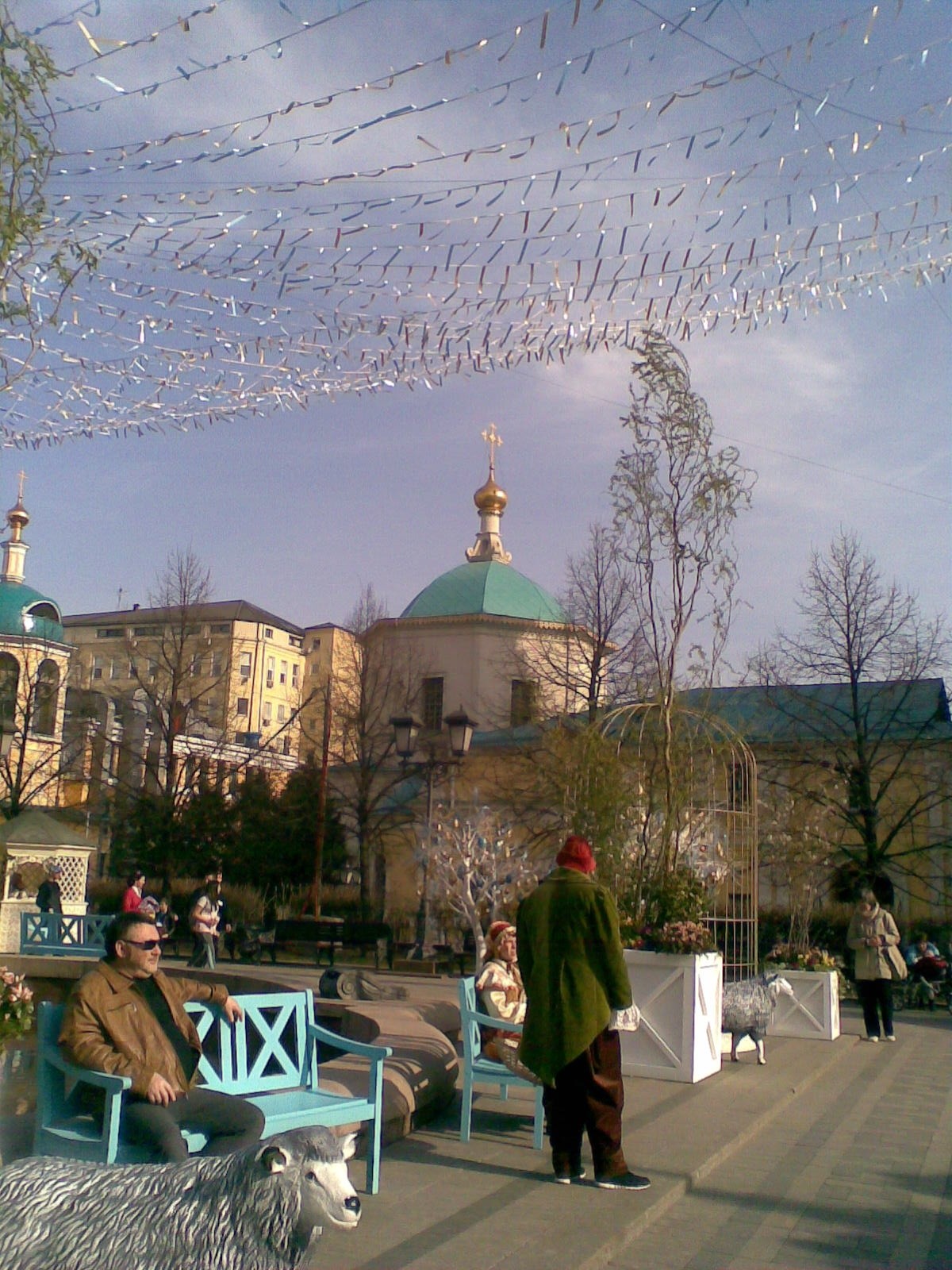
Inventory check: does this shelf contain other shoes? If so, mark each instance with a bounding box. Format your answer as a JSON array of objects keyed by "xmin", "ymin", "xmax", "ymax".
[
  {"xmin": 594, "ymin": 1170, "xmax": 651, "ymax": 1190},
  {"xmin": 868, "ymin": 1035, "xmax": 879, "ymax": 1043},
  {"xmin": 887, "ymin": 1035, "xmax": 896, "ymax": 1041},
  {"xmin": 555, "ymin": 1167, "xmax": 586, "ymax": 1185}
]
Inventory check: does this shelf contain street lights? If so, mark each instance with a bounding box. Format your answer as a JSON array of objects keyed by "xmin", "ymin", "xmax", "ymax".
[{"xmin": 388, "ymin": 702, "xmax": 480, "ymax": 962}]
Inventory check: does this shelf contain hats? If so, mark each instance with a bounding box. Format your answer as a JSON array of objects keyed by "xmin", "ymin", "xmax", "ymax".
[{"xmin": 49, "ymin": 865, "xmax": 60, "ymax": 873}]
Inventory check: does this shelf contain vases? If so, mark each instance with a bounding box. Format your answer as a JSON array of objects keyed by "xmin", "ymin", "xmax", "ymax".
[
  {"xmin": 762, "ymin": 969, "xmax": 841, "ymax": 1042},
  {"xmin": 619, "ymin": 948, "xmax": 724, "ymax": 1084}
]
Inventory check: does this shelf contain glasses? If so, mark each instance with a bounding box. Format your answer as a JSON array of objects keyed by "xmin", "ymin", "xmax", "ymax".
[{"xmin": 120, "ymin": 935, "xmax": 162, "ymax": 950}]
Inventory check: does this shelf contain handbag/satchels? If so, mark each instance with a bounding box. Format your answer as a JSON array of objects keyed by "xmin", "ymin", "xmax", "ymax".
[{"xmin": 883, "ymin": 944, "xmax": 908, "ymax": 982}]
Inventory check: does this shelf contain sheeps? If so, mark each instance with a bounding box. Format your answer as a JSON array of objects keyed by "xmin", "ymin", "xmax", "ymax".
[
  {"xmin": 0, "ymin": 1122, "xmax": 374, "ymax": 1270},
  {"xmin": 721, "ymin": 970, "xmax": 796, "ymax": 1066}
]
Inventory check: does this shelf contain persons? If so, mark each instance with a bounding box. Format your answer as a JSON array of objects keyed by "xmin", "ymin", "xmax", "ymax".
[
  {"xmin": 57, "ymin": 912, "xmax": 265, "ymax": 1162},
  {"xmin": 186, "ymin": 874, "xmax": 232, "ymax": 969},
  {"xmin": 155, "ymin": 898, "xmax": 178, "ymax": 959},
  {"xmin": 475, "ymin": 921, "xmax": 542, "ymax": 1087},
  {"xmin": 516, "ymin": 836, "xmax": 651, "ymax": 1190},
  {"xmin": 36, "ymin": 866, "xmax": 64, "ymax": 956},
  {"xmin": 906, "ymin": 932, "xmax": 952, "ymax": 1009},
  {"xmin": 847, "ymin": 889, "xmax": 900, "ymax": 1041},
  {"xmin": 121, "ymin": 870, "xmax": 156, "ymax": 919}
]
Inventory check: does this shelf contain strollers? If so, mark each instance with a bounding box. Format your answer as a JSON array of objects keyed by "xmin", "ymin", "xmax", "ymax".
[{"xmin": 892, "ymin": 943, "xmax": 952, "ymax": 1016}]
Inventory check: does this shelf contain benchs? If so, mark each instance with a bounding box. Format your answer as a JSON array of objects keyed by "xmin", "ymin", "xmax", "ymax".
[
  {"xmin": 456, "ymin": 975, "xmax": 546, "ymax": 1151},
  {"xmin": 20, "ymin": 911, "xmax": 118, "ymax": 957},
  {"xmin": 259, "ymin": 919, "xmax": 395, "ymax": 972},
  {"xmin": 160, "ymin": 917, "xmax": 237, "ymax": 963},
  {"xmin": 34, "ymin": 988, "xmax": 393, "ymax": 1196}
]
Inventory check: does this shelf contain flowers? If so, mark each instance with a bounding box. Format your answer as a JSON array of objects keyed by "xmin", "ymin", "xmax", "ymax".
[
  {"xmin": 763, "ymin": 944, "xmax": 851, "ymax": 1001},
  {"xmin": 0, "ymin": 967, "xmax": 35, "ymax": 1039},
  {"xmin": 620, "ymin": 914, "xmax": 723, "ymax": 959}
]
